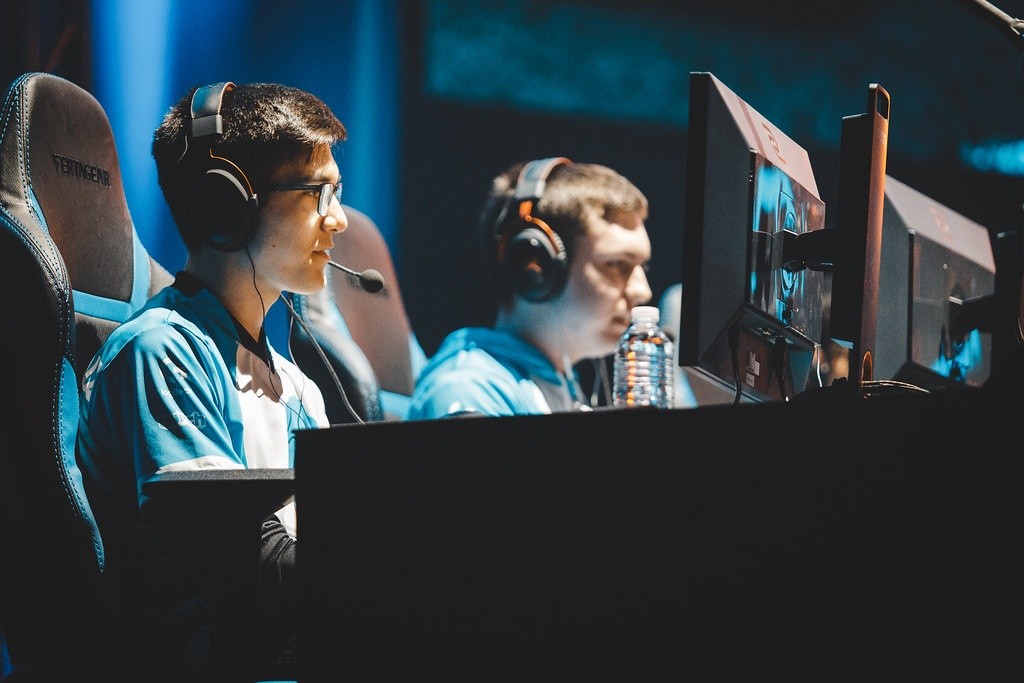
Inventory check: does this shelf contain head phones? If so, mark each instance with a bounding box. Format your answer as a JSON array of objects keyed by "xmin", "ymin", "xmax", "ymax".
[
  {"xmin": 495, "ymin": 158, "xmax": 573, "ymax": 303},
  {"xmin": 176, "ymin": 82, "xmax": 262, "ymax": 253}
]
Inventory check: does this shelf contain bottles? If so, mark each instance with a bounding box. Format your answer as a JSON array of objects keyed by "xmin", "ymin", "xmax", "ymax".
[{"xmin": 613, "ymin": 306, "xmax": 674, "ymax": 410}]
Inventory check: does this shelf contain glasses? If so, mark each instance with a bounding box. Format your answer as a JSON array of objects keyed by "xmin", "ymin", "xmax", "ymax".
[{"xmin": 269, "ymin": 181, "xmax": 343, "ymax": 216}]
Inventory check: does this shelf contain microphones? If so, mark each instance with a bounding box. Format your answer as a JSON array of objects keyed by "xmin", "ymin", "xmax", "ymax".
[{"xmin": 327, "ymin": 262, "xmax": 385, "ymax": 294}]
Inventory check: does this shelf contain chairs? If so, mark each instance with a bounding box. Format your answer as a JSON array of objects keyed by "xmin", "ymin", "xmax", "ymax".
[
  {"xmin": 0, "ymin": 72, "xmax": 177, "ymax": 667},
  {"xmin": 658, "ymin": 282, "xmax": 752, "ymax": 405},
  {"xmin": 323, "ymin": 203, "xmax": 427, "ymax": 422}
]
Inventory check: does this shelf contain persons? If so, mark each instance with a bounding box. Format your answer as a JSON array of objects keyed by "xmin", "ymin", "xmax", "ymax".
[
  {"xmin": 72, "ymin": 79, "xmax": 351, "ymax": 596},
  {"xmin": 402, "ymin": 156, "xmax": 653, "ymax": 419}
]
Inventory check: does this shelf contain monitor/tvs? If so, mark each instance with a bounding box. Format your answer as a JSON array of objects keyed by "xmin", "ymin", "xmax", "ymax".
[{"xmin": 678, "ymin": 72, "xmax": 1024, "ymax": 404}]
